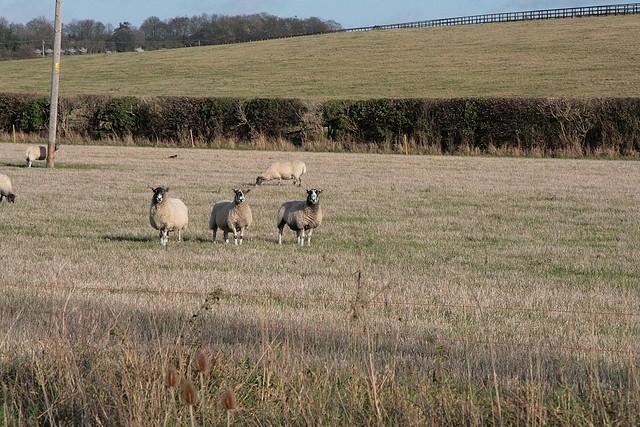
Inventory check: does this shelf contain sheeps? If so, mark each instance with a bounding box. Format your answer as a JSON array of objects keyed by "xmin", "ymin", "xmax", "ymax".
[
  {"xmin": 254, "ymin": 159, "xmax": 306, "ymax": 188},
  {"xmin": 208, "ymin": 188, "xmax": 252, "ymax": 245},
  {"xmin": 0, "ymin": 173, "xmax": 17, "ymax": 205},
  {"xmin": 149, "ymin": 187, "xmax": 188, "ymax": 246},
  {"xmin": 26, "ymin": 144, "xmax": 60, "ymax": 168},
  {"xmin": 275, "ymin": 188, "xmax": 324, "ymax": 246}
]
